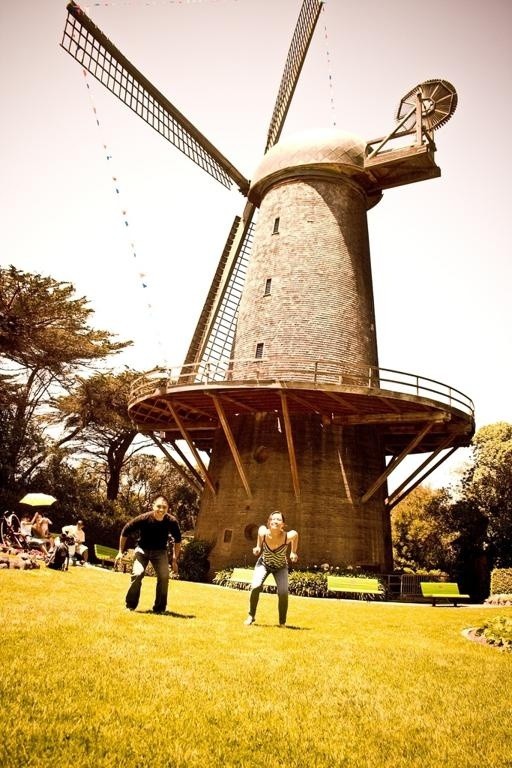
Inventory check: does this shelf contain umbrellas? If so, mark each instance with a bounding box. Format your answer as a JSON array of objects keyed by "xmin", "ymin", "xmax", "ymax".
[{"xmin": 18, "ymin": 492, "xmax": 58, "ymax": 506}]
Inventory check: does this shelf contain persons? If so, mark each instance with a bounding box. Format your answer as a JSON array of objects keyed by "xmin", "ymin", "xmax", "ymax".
[
  {"xmin": 113, "ymin": 495, "xmax": 181, "ymax": 615},
  {"xmin": 0, "ymin": 510, "xmax": 90, "ymax": 570},
  {"xmin": 244, "ymin": 511, "xmax": 299, "ymax": 628}
]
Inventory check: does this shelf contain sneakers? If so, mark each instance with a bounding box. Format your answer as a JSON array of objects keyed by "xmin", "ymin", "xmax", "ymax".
[{"xmin": 245, "ymin": 616, "xmax": 255, "ymax": 625}]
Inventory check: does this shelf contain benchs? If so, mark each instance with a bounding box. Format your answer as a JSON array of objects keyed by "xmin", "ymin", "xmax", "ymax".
[
  {"xmin": 95, "ymin": 544, "xmax": 130, "ymax": 573},
  {"xmin": 325, "ymin": 576, "xmax": 384, "ymax": 600},
  {"xmin": 420, "ymin": 582, "xmax": 470, "ymax": 607},
  {"xmin": 226, "ymin": 567, "xmax": 277, "ymax": 592}
]
